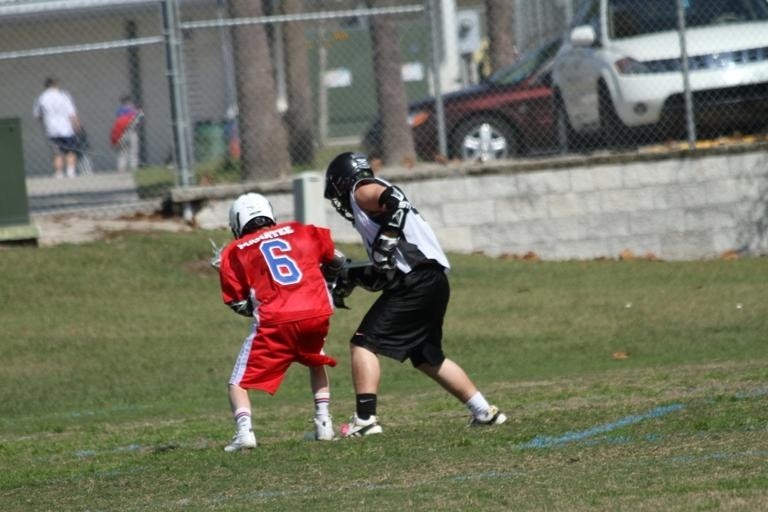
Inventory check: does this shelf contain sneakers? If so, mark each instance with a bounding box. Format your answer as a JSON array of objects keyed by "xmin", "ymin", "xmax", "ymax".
[
  {"xmin": 314, "ymin": 417, "xmax": 335, "ymax": 441},
  {"xmin": 466, "ymin": 404, "xmax": 508, "ymax": 430},
  {"xmin": 224, "ymin": 430, "xmax": 256, "ymax": 452},
  {"xmin": 332, "ymin": 411, "xmax": 383, "ymax": 439}
]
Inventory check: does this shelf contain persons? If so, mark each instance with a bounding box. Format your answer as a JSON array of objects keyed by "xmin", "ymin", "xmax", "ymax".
[
  {"xmin": 324, "ymin": 151, "xmax": 509, "ymax": 443},
  {"xmin": 111, "ymin": 92, "xmax": 145, "ymax": 175},
  {"xmin": 468, "ymin": 40, "xmax": 491, "ymax": 86},
  {"xmin": 210, "ymin": 190, "xmax": 351, "ymax": 455},
  {"xmin": 31, "ymin": 78, "xmax": 87, "ymax": 178}
]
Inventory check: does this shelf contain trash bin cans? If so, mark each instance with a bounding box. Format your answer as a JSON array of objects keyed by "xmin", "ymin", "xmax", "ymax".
[{"xmin": 194, "ymin": 112, "xmax": 315, "ymax": 180}]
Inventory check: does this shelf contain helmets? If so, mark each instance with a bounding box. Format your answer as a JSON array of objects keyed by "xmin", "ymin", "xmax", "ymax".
[
  {"xmin": 228, "ymin": 191, "xmax": 276, "ymax": 240},
  {"xmin": 324, "ymin": 151, "xmax": 375, "ymax": 214}
]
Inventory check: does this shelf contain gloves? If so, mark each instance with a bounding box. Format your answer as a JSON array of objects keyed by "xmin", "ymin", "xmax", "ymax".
[{"xmin": 229, "ymin": 225, "xmax": 406, "ymax": 318}]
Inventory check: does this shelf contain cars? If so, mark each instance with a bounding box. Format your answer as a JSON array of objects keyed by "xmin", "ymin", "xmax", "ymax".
[
  {"xmin": 362, "ymin": 34, "xmax": 568, "ymax": 165},
  {"xmin": 553, "ymin": 2, "xmax": 766, "ymax": 153}
]
